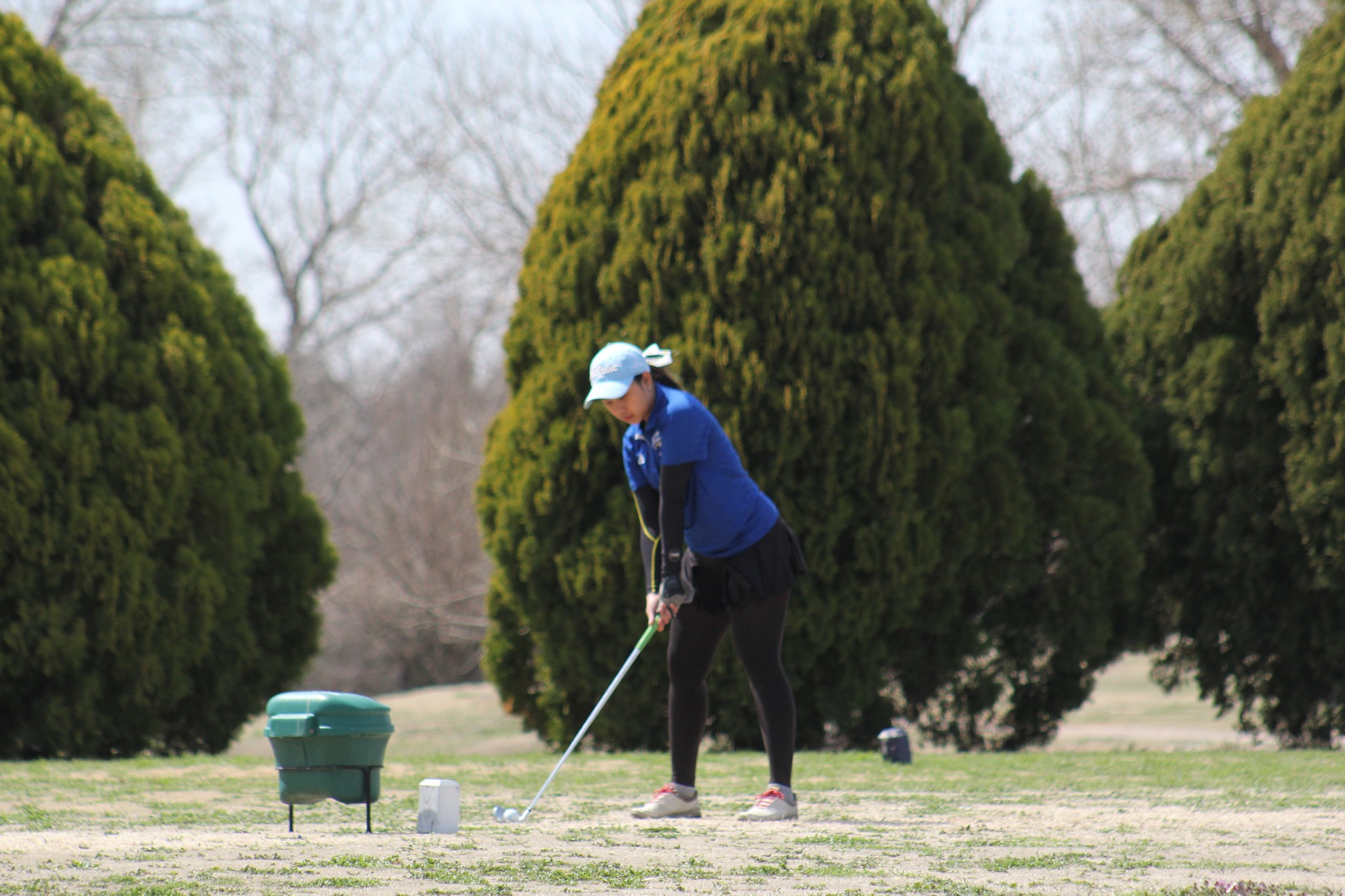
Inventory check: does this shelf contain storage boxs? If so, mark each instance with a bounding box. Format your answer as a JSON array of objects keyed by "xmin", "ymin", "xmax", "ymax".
[
  {"xmin": 266, "ymin": 690, "xmax": 394, "ymax": 804},
  {"xmin": 418, "ymin": 779, "xmax": 460, "ymax": 833}
]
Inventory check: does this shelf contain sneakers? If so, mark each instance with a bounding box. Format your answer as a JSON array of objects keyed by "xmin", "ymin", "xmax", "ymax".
[
  {"xmin": 631, "ymin": 783, "xmax": 701, "ymax": 818},
  {"xmin": 737, "ymin": 785, "xmax": 798, "ymax": 820}
]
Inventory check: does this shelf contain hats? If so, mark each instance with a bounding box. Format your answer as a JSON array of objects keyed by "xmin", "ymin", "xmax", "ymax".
[{"xmin": 583, "ymin": 341, "xmax": 651, "ymax": 410}]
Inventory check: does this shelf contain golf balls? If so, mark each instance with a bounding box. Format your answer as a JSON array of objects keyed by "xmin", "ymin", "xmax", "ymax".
[{"xmin": 503, "ymin": 808, "xmax": 518, "ymax": 822}]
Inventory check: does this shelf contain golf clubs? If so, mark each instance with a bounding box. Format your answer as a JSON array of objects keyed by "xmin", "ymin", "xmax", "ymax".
[{"xmin": 492, "ymin": 605, "xmax": 673, "ymax": 824}]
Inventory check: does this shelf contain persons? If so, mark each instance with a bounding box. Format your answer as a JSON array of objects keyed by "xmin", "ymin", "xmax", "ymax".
[{"xmin": 583, "ymin": 339, "xmax": 802, "ymax": 824}]
